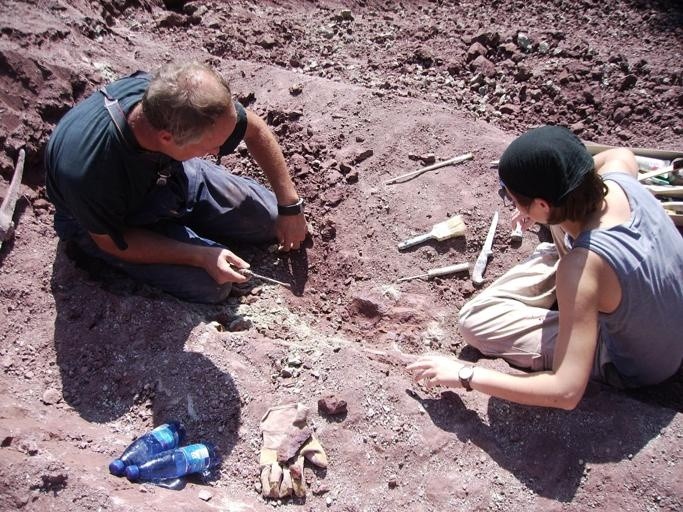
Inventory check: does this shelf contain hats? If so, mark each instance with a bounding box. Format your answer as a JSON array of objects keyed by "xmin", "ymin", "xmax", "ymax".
[{"xmin": 498, "ymin": 127, "xmax": 595, "ymax": 205}]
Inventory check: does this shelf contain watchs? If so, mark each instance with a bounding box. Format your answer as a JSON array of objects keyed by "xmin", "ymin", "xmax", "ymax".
[{"xmin": 458, "ymin": 365, "xmax": 476, "ymax": 391}]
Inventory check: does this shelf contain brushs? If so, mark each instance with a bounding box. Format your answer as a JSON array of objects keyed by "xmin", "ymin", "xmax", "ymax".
[{"xmin": 398, "ymin": 215, "xmax": 467, "ymax": 251}]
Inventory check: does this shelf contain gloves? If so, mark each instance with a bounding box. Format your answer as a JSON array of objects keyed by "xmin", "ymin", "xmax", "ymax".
[{"xmin": 260, "ymin": 404, "xmax": 328, "ymax": 499}]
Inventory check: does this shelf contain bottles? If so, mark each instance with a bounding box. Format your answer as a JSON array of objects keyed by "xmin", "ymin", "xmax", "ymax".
[
  {"xmin": 108, "ymin": 418, "xmax": 186, "ymax": 477},
  {"xmin": 123, "ymin": 440, "xmax": 219, "ymax": 482}
]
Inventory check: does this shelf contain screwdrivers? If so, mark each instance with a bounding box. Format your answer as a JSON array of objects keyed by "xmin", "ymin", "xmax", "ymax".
[
  {"xmin": 229, "ymin": 262, "xmax": 291, "ymax": 287},
  {"xmin": 399, "ymin": 262, "xmax": 470, "ymax": 281}
]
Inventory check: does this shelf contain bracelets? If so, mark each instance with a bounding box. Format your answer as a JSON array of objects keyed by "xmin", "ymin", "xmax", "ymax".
[{"xmin": 277, "ymin": 199, "xmax": 306, "ymax": 216}]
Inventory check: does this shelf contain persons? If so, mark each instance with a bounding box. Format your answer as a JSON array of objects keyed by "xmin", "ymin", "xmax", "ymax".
[
  {"xmin": 45, "ymin": 59, "xmax": 309, "ymax": 304},
  {"xmin": 405, "ymin": 126, "xmax": 683, "ymax": 412}
]
